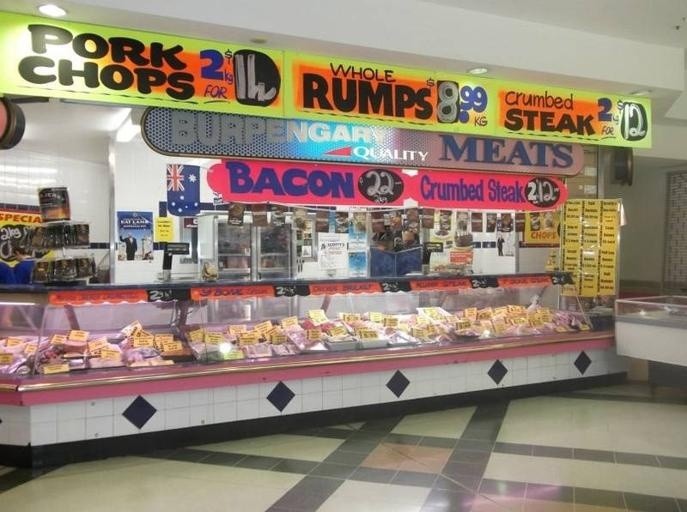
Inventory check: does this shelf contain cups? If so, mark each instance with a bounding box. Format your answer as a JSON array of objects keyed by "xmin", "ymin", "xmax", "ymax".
[{"xmin": 242, "ymin": 304, "xmax": 252, "ymax": 321}]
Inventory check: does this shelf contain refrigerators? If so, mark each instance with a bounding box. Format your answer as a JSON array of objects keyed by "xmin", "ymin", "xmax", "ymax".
[{"xmin": 197, "ymin": 210, "xmax": 299, "ymax": 319}]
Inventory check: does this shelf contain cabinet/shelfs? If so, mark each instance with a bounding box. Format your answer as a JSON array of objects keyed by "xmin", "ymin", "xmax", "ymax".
[
  {"xmin": 0, "ymin": 272, "xmax": 629, "ymax": 446},
  {"xmin": 614, "ymin": 297, "xmax": 687, "ymax": 369}
]
